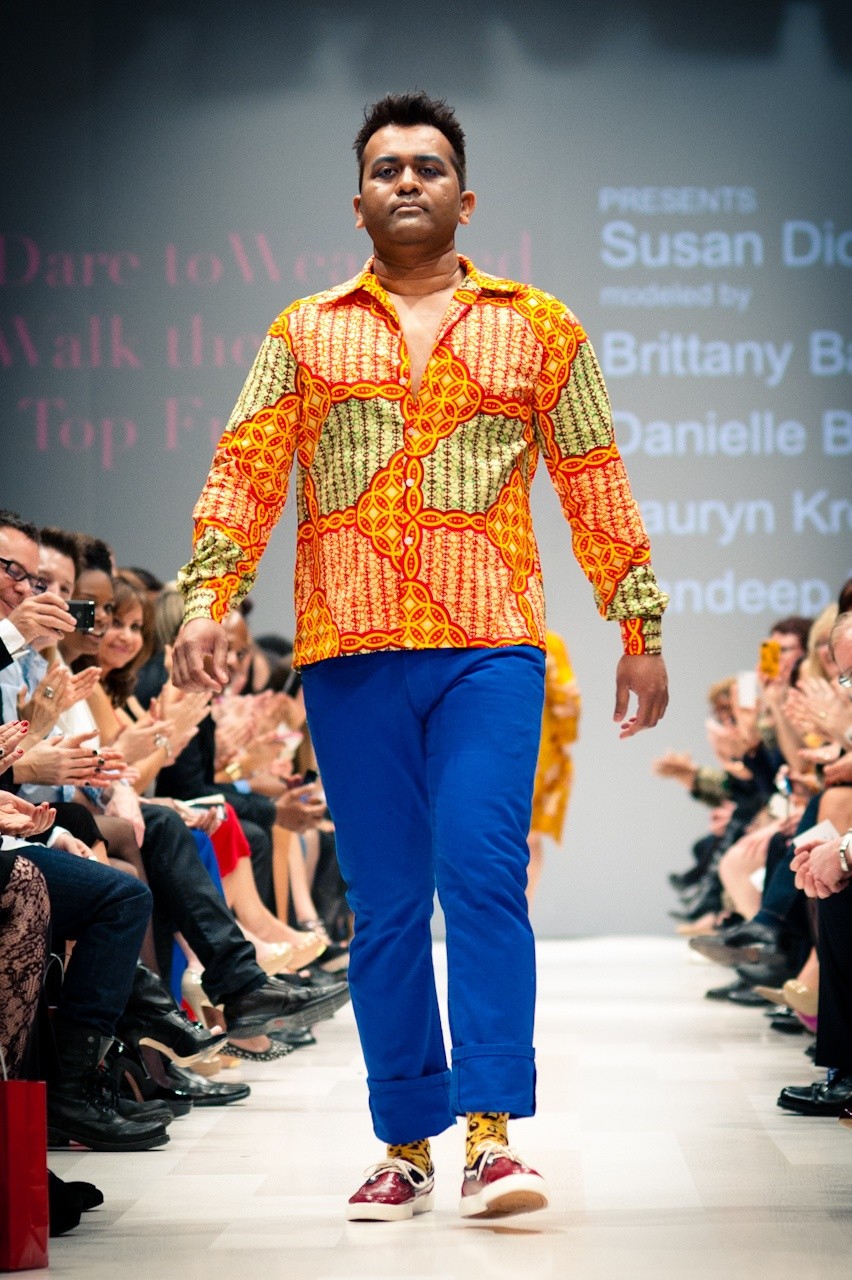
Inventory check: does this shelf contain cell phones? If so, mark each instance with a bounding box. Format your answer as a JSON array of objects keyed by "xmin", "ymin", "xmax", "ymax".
[{"xmin": 64, "ymin": 599, "xmax": 95, "ymax": 628}]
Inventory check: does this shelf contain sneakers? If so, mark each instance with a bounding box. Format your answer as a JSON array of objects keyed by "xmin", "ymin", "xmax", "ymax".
[
  {"xmin": 346, "ymin": 1159, "xmax": 435, "ymax": 1223},
  {"xmin": 459, "ymin": 1141, "xmax": 548, "ymax": 1221}
]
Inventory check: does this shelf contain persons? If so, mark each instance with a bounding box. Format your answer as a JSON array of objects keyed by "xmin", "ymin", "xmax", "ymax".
[
  {"xmin": 515, "ymin": 617, "xmax": 584, "ymax": 924},
  {"xmin": 656, "ymin": 574, "xmax": 852, "ymax": 1124},
  {"xmin": 160, "ymin": 86, "xmax": 667, "ymax": 1225},
  {"xmin": 0, "ymin": 509, "xmax": 357, "ymax": 1237}
]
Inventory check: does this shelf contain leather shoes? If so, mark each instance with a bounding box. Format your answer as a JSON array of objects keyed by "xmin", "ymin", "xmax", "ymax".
[
  {"xmin": 668, "ymin": 867, "xmax": 852, "ymax": 1119},
  {"xmin": 19, "ymin": 920, "xmax": 351, "ymax": 1237}
]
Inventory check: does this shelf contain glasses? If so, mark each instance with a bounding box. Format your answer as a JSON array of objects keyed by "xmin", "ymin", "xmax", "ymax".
[
  {"xmin": 837, "ymin": 673, "xmax": 852, "ymax": 689},
  {"xmin": 0, "ymin": 556, "xmax": 49, "ymax": 596}
]
